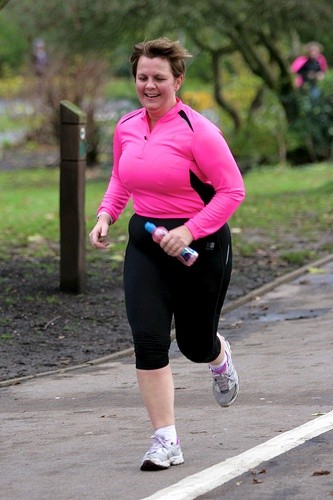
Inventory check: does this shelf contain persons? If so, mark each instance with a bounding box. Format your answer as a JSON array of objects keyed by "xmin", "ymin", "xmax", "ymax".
[
  {"xmin": 87, "ymin": 37, "xmax": 245, "ymax": 471},
  {"xmin": 289, "ymin": 41, "xmax": 328, "ymax": 102}
]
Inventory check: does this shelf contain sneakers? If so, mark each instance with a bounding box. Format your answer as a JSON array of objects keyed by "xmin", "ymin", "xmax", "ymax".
[
  {"xmin": 210, "ymin": 337, "xmax": 239, "ymax": 408},
  {"xmin": 140, "ymin": 434, "xmax": 184, "ymax": 471}
]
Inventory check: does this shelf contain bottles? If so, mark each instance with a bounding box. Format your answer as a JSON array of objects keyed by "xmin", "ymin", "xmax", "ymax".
[{"xmin": 145, "ymin": 222, "xmax": 199, "ymax": 267}]
